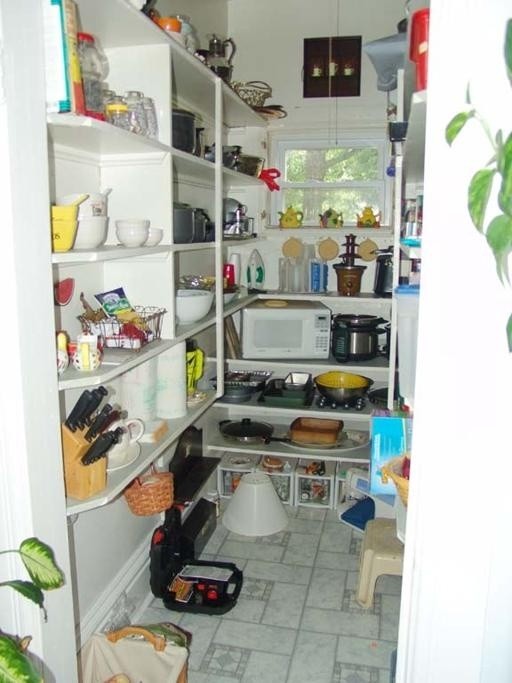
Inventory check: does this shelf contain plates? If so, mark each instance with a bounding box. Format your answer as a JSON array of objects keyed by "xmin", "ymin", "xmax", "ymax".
[
  {"xmin": 107, "ymin": 442, "xmax": 140, "ymax": 473},
  {"xmin": 263, "ymin": 460, "xmax": 284, "ymax": 468},
  {"xmin": 228, "ymin": 455, "xmax": 251, "ymax": 467},
  {"xmin": 280, "ymin": 428, "xmax": 368, "ymax": 452},
  {"xmin": 186, "ymin": 397, "xmax": 206, "ymax": 407}
]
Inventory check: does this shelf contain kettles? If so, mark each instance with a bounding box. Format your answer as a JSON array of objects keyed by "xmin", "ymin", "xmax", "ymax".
[{"xmin": 205, "ymin": 33, "xmax": 236, "ymax": 81}]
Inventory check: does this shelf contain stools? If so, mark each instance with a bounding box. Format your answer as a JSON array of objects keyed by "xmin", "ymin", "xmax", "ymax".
[{"xmin": 355, "ymin": 519, "xmax": 405, "ymax": 611}]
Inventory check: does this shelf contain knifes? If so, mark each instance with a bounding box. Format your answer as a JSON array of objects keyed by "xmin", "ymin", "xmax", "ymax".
[{"xmin": 65, "ymin": 385, "xmax": 122, "ymax": 464}]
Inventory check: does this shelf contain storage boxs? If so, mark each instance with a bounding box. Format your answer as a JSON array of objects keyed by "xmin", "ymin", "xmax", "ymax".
[
  {"xmin": 395, "ymin": 280, "xmax": 418, "ymax": 400},
  {"xmin": 216, "ymin": 452, "xmax": 367, "ymax": 511},
  {"xmin": 147, "ymin": 504, "xmax": 243, "ymax": 614}
]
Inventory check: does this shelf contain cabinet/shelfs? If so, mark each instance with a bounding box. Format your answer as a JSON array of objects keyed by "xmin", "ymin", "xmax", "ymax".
[
  {"xmin": 204, "ymin": 287, "xmax": 394, "ymax": 466},
  {"xmin": 384, "ymin": 66, "xmax": 426, "ymax": 422},
  {"xmin": 45, "ymin": 0, "xmax": 271, "ymax": 514}
]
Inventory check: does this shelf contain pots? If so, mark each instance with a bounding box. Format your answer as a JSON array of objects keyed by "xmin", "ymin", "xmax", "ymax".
[
  {"xmin": 172, "ymin": 202, "xmax": 216, "ymax": 244},
  {"xmin": 218, "ymin": 417, "xmax": 275, "ymax": 445},
  {"xmin": 172, "ymin": 108, "xmax": 205, "ymax": 159},
  {"xmin": 314, "ymin": 374, "xmax": 374, "ymax": 402}
]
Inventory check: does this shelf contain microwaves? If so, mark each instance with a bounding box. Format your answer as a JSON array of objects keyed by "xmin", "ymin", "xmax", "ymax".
[{"xmin": 240, "ymin": 298, "xmax": 331, "ymax": 359}]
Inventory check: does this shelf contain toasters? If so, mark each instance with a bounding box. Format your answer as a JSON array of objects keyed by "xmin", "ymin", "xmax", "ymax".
[{"xmin": 371, "ymin": 245, "xmax": 393, "ymax": 294}]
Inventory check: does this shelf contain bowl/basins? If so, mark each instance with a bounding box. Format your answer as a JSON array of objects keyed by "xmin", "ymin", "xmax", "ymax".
[
  {"xmin": 386, "ymin": 454, "xmax": 411, "ymax": 506},
  {"xmin": 241, "ymin": 154, "xmax": 266, "ymax": 178},
  {"xmin": 153, "ymin": 17, "xmax": 182, "ymax": 33},
  {"xmin": 174, "ymin": 287, "xmax": 214, "ymax": 326},
  {"xmin": 50, "ymin": 190, "xmax": 109, "ymax": 253},
  {"xmin": 114, "ymin": 217, "xmax": 165, "ymax": 247},
  {"xmin": 222, "ymin": 151, "xmax": 238, "ymax": 167},
  {"xmin": 222, "ymin": 292, "xmax": 236, "ymax": 305},
  {"xmin": 232, "ymin": 84, "xmax": 272, "ymax": 109}
]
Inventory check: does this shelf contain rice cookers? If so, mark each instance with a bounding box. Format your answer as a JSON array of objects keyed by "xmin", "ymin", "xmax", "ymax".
[{"xmin": 329, "ymin": 311, "xmax": 388, "ymax": 361}]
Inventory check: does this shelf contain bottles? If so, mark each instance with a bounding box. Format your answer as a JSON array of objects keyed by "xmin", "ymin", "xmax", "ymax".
[
  {"xmin": 224, "ymin": 471, "xmax": 241, "ymax": 495},
  {"xmin": 75, "ymin": 32, "xmax": 159, "ymax": 138},
  {"xmin": 246, "ymin": 248, "xmax": 266, "ymax": 289},
  {"xmin": 224, "ymin": 252, "xmax": 241, "ymax": 286}
]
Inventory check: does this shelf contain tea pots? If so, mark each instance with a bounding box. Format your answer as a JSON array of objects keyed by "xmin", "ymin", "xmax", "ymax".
[
  {"xmin": 107, "ymin": 417, "xmax": 146, "ymax": 456},
  {"xmin": 355, "ymin": 205, "xmax": 382, "ymax": 227},
  {"xmin": 317, "ymin": 207, "xmax": 344, "ymax": 227},
  {"xmin": 277, "ymin": 205, "xmax": 304, "ymax": 228}
]
